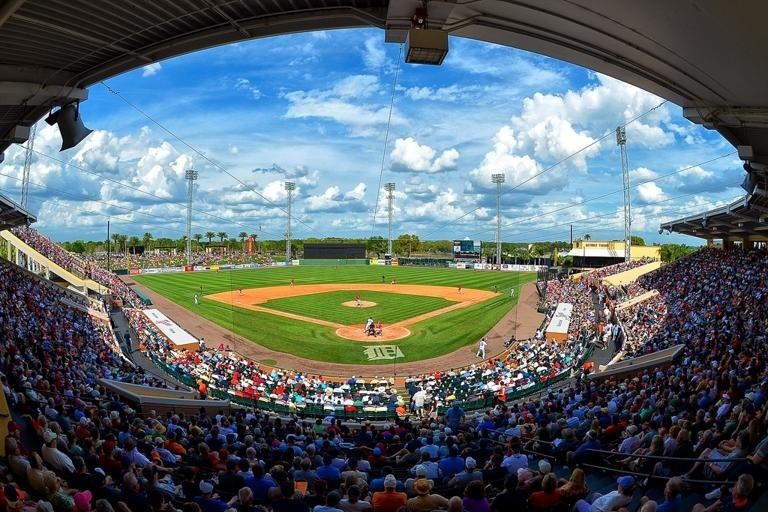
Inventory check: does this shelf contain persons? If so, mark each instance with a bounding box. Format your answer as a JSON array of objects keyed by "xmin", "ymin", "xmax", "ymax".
[{"xmin": 1, "ymin": 223, "xmax": 768, "ymax": 512}]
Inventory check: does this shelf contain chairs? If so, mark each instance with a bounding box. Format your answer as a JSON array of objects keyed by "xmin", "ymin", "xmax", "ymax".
[{"xmin": 73, "ymin": 255, "xmax": 273, "ymax": 270}]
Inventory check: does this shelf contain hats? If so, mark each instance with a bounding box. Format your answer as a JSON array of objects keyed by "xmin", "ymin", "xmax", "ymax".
[
  {"xmin": 5, "ymin": 408, "xmax": 167, "ymax": 451},
  {"xmin": 384, "ymin": 425, "xmax": 639, "ymax": 495}
]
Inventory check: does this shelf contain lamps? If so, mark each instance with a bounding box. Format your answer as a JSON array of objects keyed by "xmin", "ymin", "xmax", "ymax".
[{"xmin": 404, "ymin": 6, "xmax": 448, "ymax": 65}]
